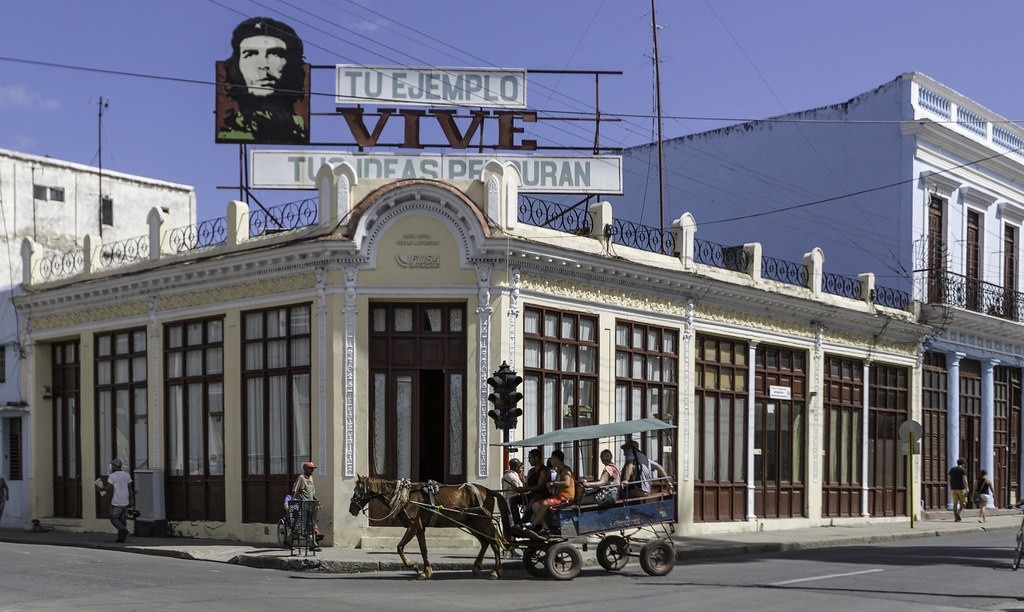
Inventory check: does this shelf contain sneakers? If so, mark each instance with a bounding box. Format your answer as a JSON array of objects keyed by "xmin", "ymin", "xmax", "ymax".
[
  {"xmin": 522, "ymin": 522, "xmax": 533, "ymax": 529},
  {"xmin": 537, "ymin": 528, "xmax": 553, "ymax": 536}
]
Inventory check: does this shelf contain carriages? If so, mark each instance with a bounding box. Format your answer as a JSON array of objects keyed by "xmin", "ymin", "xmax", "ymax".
[{"xmin": 348, "ymin": 418, "xmax": 680, "ymax": 581}]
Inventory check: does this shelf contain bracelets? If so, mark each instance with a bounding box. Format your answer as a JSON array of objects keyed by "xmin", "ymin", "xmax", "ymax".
[
  {"xmin": 550, "ymin": 482, "xmax": 554, "ymax": 486},
  {"xmin": 623, "ymin": 479, "xmax": 629, "ymax": 481}
]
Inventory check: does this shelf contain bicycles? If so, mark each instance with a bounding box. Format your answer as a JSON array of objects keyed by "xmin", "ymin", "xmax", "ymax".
[{"xmin": 1012, "ymin": 514, "xmax": 1024, "ymax": 571}]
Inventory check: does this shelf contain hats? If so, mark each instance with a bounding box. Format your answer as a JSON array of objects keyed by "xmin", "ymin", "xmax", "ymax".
[
  {"xmin": 303, "ymin": 462, "xmax": 317, "ymax": 468},
  {"xmin": 621, "ymin": 441, "xmax": 639, "ymax": 449},
  {"xmin": 601, "ymin": 450, "xmax": 612, "ymax": 459},
  {"xmin": 109, "ymin": 459, "xmax": 122, "ymax": 467}
]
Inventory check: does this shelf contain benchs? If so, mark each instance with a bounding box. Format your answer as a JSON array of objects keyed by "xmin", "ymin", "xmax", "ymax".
[{"xmin": 573, "ymin": 490, "xmax": 679, "ymax": 509}]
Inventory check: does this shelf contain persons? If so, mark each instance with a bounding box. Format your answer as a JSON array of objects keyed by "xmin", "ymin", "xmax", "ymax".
[
  {"xmin": 580, "ymin": 449, "xmax": 620, "ymax": 505},
  {"xmin": 522, "ymin": 450, "xmax": 575, "ymax": 535},
  {"xmin": 502, "ymin": 459, "xmax": 525, "ymax": 559},
  {"xmin": 546, "ymin": 458, "xmax": 558, "ymax": 482},
  {"xmin": 948, "ymin": 458, "xmax": 995, "ymax": 523},
  {"xmin": 509, "ymin": 449, "xmax": 551, "ymax": 530},
  {"xmin": 283, "ymin": 492, "xmax": 325, "ymax": 540},
  {"xmin": 219, "ymin": 16, "xmax": 307, "ymax": 138},
  {"xmin": 97, "ymin": 459, "xmax": 135, "ymax": 543},
  {"xmin": 0, "ymin": 477, "xmax": 9, "ymax": 519},
  {"xmin": 285, "ymin": 462, "xmax": 322, "ymax": 551},
  {"xmin": 618, "ymin": 441, "xmax": 674, "ymax": 499}
]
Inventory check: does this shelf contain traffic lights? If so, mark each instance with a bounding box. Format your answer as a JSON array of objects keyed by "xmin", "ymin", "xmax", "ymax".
[
  {"xmin": 504, "ymin": 374, "xmax": 523, "ymax": 422},
  {"xmin": 487, "ymin": 375, "xmax": 503, "ymax": 422}
]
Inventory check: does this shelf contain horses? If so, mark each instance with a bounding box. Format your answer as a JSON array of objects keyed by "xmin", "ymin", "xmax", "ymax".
[{"xmin": 349, "ymin": 473, "xmax": 511, "ymax": 580}]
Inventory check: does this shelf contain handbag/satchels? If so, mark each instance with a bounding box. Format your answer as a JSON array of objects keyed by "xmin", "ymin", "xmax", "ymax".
[
  {"xmin": 593, "ymin": 485, "xmax": 619, "ymax": 504},
  {"xmin": 621, "ymin": 448, "xmax": 642, "ymax": 491}
]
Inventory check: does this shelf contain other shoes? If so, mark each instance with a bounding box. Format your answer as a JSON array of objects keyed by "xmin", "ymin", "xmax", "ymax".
[
  {"xmin": 309, "ymin": 547, "xmax": 322, "ymax": 551},
  {"xmin": 955, "ymin": 512, "xmax": 962, "ymax": 521},
  {"xmin": 978, "ymin": 519, "xmax": 983, "ymax": 523},
  {"xmin": 115, "ymin": 529, "xmax": 129, "ymax": 543}
]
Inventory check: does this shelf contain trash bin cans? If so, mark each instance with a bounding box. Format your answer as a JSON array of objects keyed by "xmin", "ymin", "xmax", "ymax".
[{"xmin": 288, "ymin": 499, "xmax": 319, "ymax": 556}]
